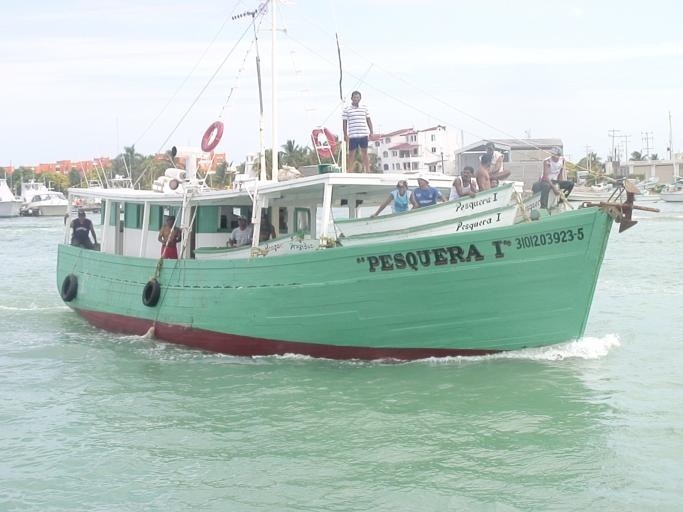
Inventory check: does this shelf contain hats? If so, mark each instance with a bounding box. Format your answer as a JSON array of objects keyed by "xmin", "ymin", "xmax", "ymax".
[
  {"xmin": 416, "ymin": 173, "xmax": 430, "ymax": 183},
  {"xmin": 548, "ymin": 146, "xmax": 562, "ymax": 155},
  {"xmin": 238, "ymin": 215, "xmax": 247, "ymax": 219}
]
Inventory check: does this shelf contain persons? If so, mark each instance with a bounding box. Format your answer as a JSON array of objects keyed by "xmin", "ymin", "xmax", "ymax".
[
  {"xmin": 225, "ymin": 214, "xmax": 253, "ymax": 249},
  {"xmin": 475, "ymin": 154, "xmax": 494, "ymax": 192},
  {"xmin": 490, "ymin": 177, "xmax": 499, "ymax": 189},
  {"xmin": 158, "ymin": 216, "xmax": 182, "ymax": 259},
  {"xmin": 413, "ymin": 176, "xmax": 447, "ymax": 210},
  {"xmin": 478, "ymin": 141, "xmax": 505, "ymax": 174},
  {"xmin": 69, "ymin": 208, "xmax": 98, "ymax": 251},
  {"xmin": 340, "ymin": 90, "xmax": 375, "ymax": 173},
  {"xmin": 531, "ymin": 146, "xmax": 574, "ymax": 211},
  {"xmin": 370, "ymin": 179, "xmax": 420, "ymax": 219},
  {"xmin": 448, "ymin": 166, "xmax": 479, "ymax": 202},
  {"xmin": 259, "ymin": 212, "xmax": 276, "ymax": 244}
]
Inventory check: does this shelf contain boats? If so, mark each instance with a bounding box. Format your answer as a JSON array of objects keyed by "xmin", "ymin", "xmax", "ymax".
[
  {"xmin": 52, "ymin": 6, "xmax": 650, "ymax": 363},
  {"xmin": 0, "ymin": 178, "xmax": 69, "ymax": 216},
  {"xmin": 658, "ymin": 177, "xmax": 683, "ymax": 201}
]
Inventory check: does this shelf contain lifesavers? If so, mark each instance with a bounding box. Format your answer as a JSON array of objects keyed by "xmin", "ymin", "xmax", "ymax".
[
  {"xmin": 311, "ymin": 126, "xmax": 336, "ymax": 157},
  {"xmin": 201, "ymin": 121, "xmax": 224, "ymax": 152}
]
